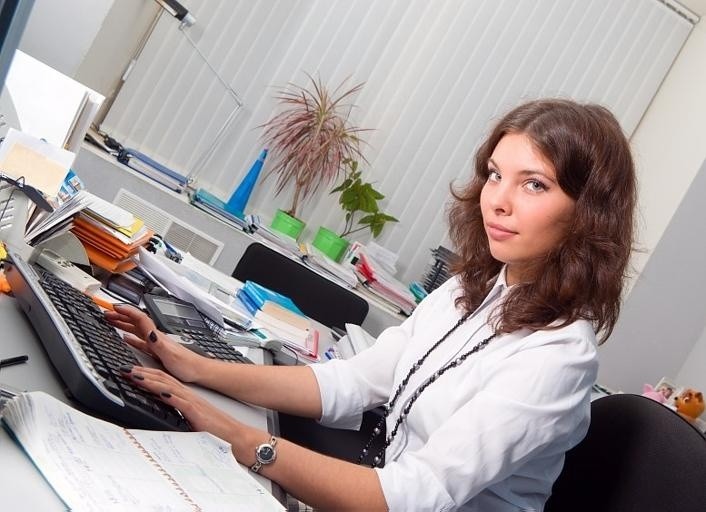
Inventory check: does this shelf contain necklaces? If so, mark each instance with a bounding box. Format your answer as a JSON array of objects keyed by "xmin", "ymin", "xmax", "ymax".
[{"xmin": 352, "ymin": 285, "xmax": 514, "ymax": 471}]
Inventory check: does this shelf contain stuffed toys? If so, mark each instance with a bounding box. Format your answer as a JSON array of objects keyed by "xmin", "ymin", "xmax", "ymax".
[
  {"xmin": 640, "ymin": 382, "xmax": 673, "ymax": 404},
  {"xmin": 673, "ymin": 388, "xmax": 706, "ymax": 424}
]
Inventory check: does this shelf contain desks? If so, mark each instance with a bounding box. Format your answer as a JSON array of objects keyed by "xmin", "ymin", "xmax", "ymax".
[{"xmin": 0, "ymin": 245, "xmax": 383, "ymax": 512}]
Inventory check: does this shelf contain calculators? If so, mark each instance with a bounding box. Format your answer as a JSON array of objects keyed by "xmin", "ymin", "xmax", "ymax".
[{"xmin": 142, "ymin": 292, "xmax": 254, "ymax": 364}]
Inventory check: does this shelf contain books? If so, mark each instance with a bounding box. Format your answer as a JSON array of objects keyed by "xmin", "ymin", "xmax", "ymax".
[
  {"xmin": 0, "ymin": 388, "xmax": 289, "ymax": 512},
  {"xmin": 242, "ymin": 278, "xmax": 311, "ymax": 331},
  {"xmin": 188, "ymin": 186, "xmax": 246, "ymax": 233},
  {"xmin": 117, "ymin": 148, "xmax": 190, "ymax": 194},
  {"xmin": 0, "ymin": 128, "xmax": 78, "ymax": 198},
  {"xmin": 242, "ymin": 213, "xmax": 420, "ymax": 320},
  {"xmin": 235, "ymin": 289, "xmax": 308, "ymax": 340}
]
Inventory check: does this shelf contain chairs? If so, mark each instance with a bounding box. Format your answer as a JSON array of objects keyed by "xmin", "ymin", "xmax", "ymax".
[{"xmin": 544, "ymin": 394, "xmax": 706, "ymax": 512}]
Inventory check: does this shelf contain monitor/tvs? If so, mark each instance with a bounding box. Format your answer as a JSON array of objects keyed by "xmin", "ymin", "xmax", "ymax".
[{"xmin": 0, "ymin": 0, "xmax": 36, "ymax": 97}]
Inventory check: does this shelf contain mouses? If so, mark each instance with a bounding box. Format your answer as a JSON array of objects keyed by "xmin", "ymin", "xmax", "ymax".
[{"xmin": 165, "ymin": 333, "xmax": 212, "ymax": 359}]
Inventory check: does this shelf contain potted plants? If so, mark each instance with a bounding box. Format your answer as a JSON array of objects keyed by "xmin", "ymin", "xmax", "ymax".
[
  {"xmin": 248, "ymin": 70, "xmax": 380, "ymax": 241},
  {"xmin": 312, "ymin": 157, "xmax": 400, "ymax": 263}
]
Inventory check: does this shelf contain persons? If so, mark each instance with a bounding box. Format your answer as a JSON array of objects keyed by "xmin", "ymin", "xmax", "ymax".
[{"xmin": 95, "ymin": 95, "xmax": 639, "ymax": 511}]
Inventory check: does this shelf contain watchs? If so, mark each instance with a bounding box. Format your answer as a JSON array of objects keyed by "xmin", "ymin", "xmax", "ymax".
[{"xmin": 250, "ymin": 432, "xmax": 278, "ymax": 475}]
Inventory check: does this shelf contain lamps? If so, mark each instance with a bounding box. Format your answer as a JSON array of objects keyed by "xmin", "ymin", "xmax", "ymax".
[{"xmin": 155, "ymin": 0, "xmax": 243, "ymax": 194}]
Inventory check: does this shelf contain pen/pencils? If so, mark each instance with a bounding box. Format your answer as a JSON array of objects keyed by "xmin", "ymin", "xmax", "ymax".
[
  {"xmin": 0, "ymin": 355, "xmax": 28, "ymax": 368},
  {"xmin": 224, "ymin": 318, "xmax": 246, "ymax": 332}
]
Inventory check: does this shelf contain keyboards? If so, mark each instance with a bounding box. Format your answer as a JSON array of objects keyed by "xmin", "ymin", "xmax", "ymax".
[{"xmin": 3, "ymin": 250, "xmax": 193, "ymax": 432}]
